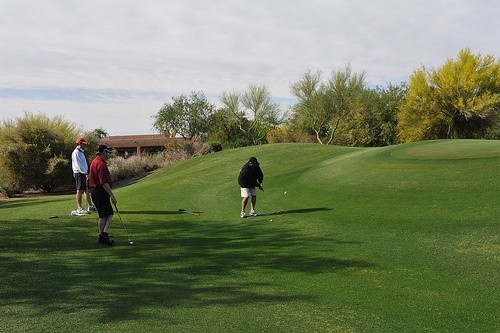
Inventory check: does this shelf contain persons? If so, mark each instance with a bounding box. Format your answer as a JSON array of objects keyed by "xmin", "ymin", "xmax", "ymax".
[
  {"xmin": 238, "ymin": 157, "xmax": 264, "ymax": 217},
  {"xmin": 88, "ymin": 144, "xmax": 117, "ymax": 245},
  {"xmin": 71, "ymin": 138, "xmax": 98, "ymax": 214}
]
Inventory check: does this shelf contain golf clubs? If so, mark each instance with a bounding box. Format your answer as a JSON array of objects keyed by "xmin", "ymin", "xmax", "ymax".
[
  {"xmin": 262, "ymin": 189, "xmax": 280, "ymax": 214},
  {"xmin": 114, "ymin": 203, "xmax": 134, "ymax": 244}
]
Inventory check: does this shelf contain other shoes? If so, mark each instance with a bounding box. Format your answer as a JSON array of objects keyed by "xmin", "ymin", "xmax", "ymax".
[
  {"xmin": 88, "ymin": 204, "xmax": 97, "ymax": 210},
  {"xmin": 98, "ymin": 232, "xmax": 114, "ymax": 246},
  {"xmin": 240, "ymin": 211, "xmax": 246, "ymax": 217},
  {"xmin": 250, "ymin": 211, "xmax": 258, "ymax": 216},
  {"xmin": 76, "ymin": 208, "xmax": 89, "ymax": 214}
]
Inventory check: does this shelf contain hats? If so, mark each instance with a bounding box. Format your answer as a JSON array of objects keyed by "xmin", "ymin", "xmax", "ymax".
[
  {"xmin": 97, "ymin": 145, "xmax": 112, "ymax": 154},
  {"xmin": 249, "ymin": 157, "xmax": 260, "ymax": 166},
  {"xmin": 76, "ymin": 137, "xmax": 89, "ymax": 145}
]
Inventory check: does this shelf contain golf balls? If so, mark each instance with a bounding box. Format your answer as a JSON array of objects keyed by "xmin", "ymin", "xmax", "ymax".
[
  {"xmin": 270, "ymin": 219, "xmax": 273, "ymax": 222},
  {"xmin": 284, "ymin": 190, "xmax": 287, "ymax": 194}
]
